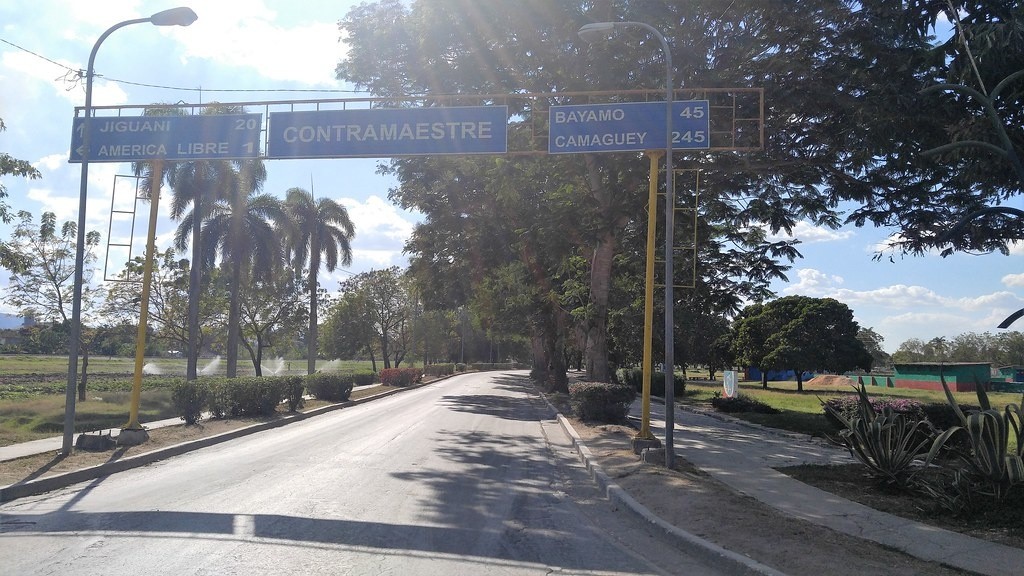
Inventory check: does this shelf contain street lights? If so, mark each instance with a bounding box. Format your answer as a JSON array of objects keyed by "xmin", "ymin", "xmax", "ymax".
[
  {"xmin": 62, "ymin": 6, "xmax": 200, "ymax": 455},
  {"xmin": 577, "ymin": 21, "xmax": 678, "ymax": 469}
]
